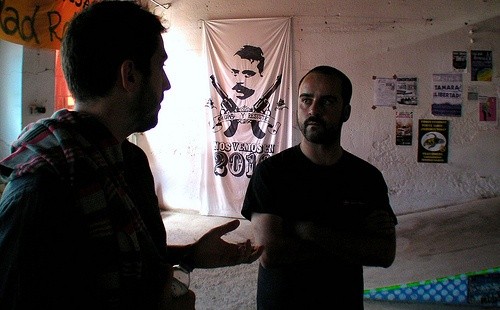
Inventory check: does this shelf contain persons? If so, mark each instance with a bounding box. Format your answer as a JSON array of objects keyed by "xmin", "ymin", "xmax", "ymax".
[
  {"xmin": 1, "ymin": 0, "xmax": 264, "ymax": 310},
  {"xmin": 241, "ymin": 65, "xmax": 399, "ymax": 310}
]
